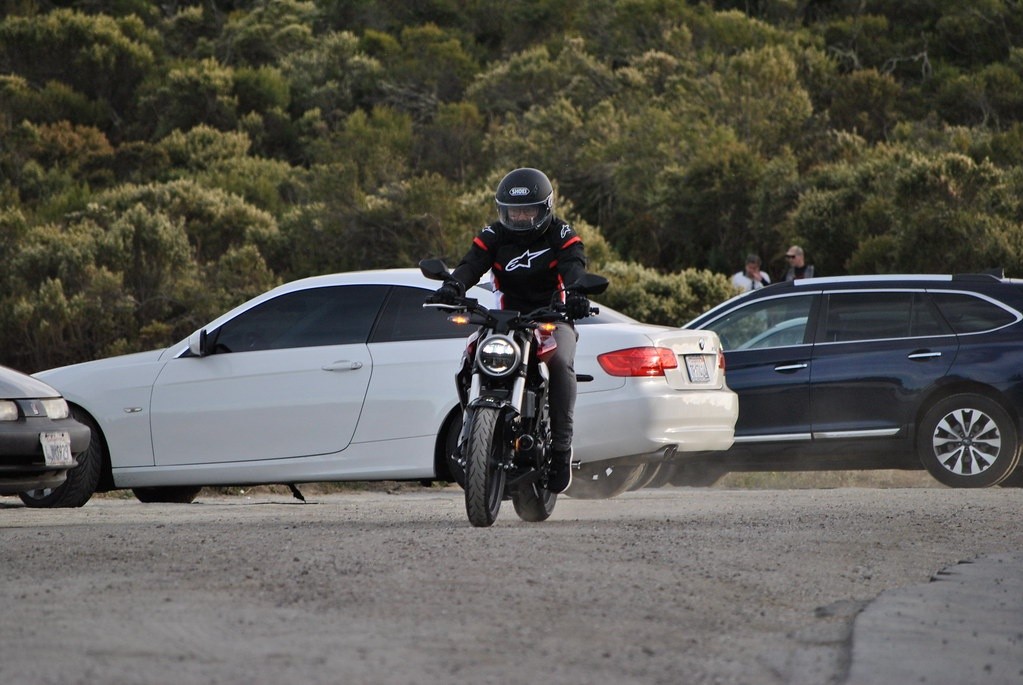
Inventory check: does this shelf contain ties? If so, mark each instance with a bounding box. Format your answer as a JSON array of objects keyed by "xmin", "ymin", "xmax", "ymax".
[{"xmin": 752, "ymin": 281, "xmax": 754, "ymax": 290}]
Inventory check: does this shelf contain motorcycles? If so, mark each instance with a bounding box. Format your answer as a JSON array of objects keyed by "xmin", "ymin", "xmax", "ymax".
[{"xmin": 419, "ymin": 260, "xmax": 610, "ymax": 529}]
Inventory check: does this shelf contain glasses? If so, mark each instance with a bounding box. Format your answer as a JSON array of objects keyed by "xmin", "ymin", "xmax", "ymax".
[
  {"xmin": 506, "ymin": 206, "xmax": 538, "ymax": 217},
  {"xmin": 786, "ymin": 255, "xmax": 796, "ymax": 259}
]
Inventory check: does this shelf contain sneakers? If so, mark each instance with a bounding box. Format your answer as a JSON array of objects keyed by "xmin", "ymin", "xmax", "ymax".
[
  {"xmin": 547, "ymin": 446, "xmax": 573, "ymax": 493},
  {"xmin": 451, "ymin": 443, "xmax": 462, "ymax": 460}
]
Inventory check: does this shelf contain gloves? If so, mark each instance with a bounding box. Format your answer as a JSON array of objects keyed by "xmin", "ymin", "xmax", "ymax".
[
  {"xmin": 433, "ymin": 280, "xmax": 465, "ymax": 313},
  {"xmin": 565, "ymin": 293, "xmax": 589, "ymax": 322}
]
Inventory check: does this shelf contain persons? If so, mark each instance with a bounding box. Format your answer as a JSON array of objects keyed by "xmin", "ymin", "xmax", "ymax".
[
  {"xmin": 432, "ymin": 167, "xmax": 590, "ymax": 494},
  {"xmin": 778, "ymin": 245, "xmax": 819, "ymax": 322},
  {"xmin": 731, "ymin": 254, "xmax": 773, "ymax": 337}
]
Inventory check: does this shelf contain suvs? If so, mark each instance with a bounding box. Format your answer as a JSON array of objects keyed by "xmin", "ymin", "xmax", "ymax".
[{"xmin": 627, "ymin": 271, "xmax": 1022, "ymax": 491}]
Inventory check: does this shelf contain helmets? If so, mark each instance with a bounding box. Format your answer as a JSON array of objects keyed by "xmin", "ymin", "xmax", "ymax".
[{"xmin": 495, "ymin": 168, "xmax": 554, "ymax": 245}]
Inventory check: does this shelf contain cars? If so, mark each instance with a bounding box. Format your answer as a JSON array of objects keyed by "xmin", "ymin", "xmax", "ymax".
[
  {"xmin": 27, "ymin": 267, "xmax": 738, "ymax": 503},
  {"xmin": 0, "ymin": 367, "xmax": 92, "ymax": 495}
]
